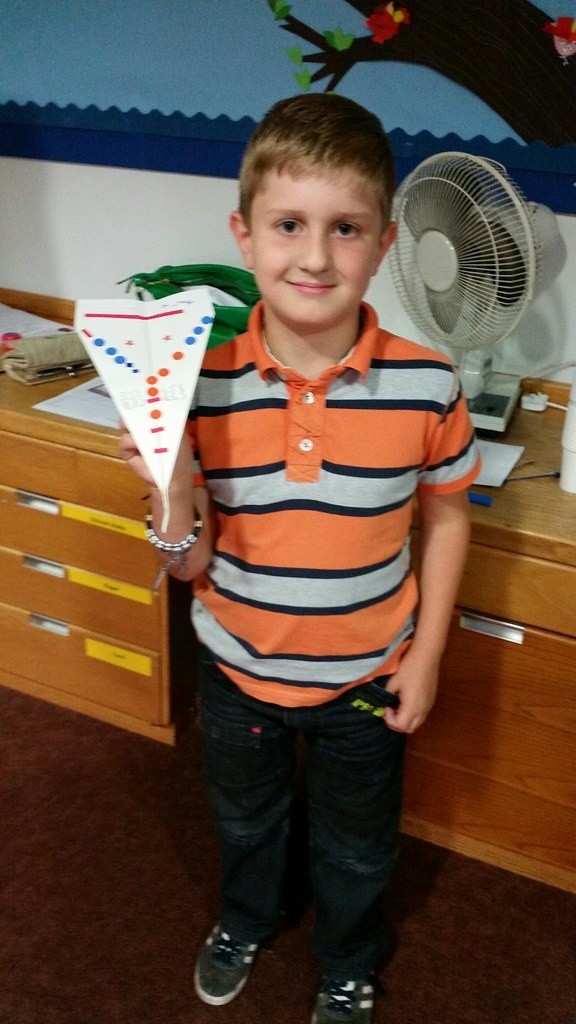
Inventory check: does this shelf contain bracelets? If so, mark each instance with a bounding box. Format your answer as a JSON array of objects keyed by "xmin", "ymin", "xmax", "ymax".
[{"xmin": 143, "ymin": 503, "xmax": 203, "ymax": 553}]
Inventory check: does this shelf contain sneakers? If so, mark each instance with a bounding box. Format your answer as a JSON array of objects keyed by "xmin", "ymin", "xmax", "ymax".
[
  {"xmin": 311, "ymin": 976, "xmax": 375, "ymax": 1023},
  {"xmin": 193, "ymin": 920, "xmax": 257, "ymax": 1005}
]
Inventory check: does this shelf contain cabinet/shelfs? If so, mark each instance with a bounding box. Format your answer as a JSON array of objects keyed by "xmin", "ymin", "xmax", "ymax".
[
  {"xmin": 402, "ymin": 379, "xmax": 574, "ymax": 896},
  {"xmin": 1, "ymin": 372, "xmax": 194, "ymax": 748}
]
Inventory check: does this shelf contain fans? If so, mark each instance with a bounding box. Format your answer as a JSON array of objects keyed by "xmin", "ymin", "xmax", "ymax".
[{"xmin": 389, "ymin": 151, "xmax": 565, "ymax": 436}]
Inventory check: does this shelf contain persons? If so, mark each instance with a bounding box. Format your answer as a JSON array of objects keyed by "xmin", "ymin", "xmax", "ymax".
[{"xmin": 120, "ymin": 91, "xmax": 482, "ymax": 1024}]
